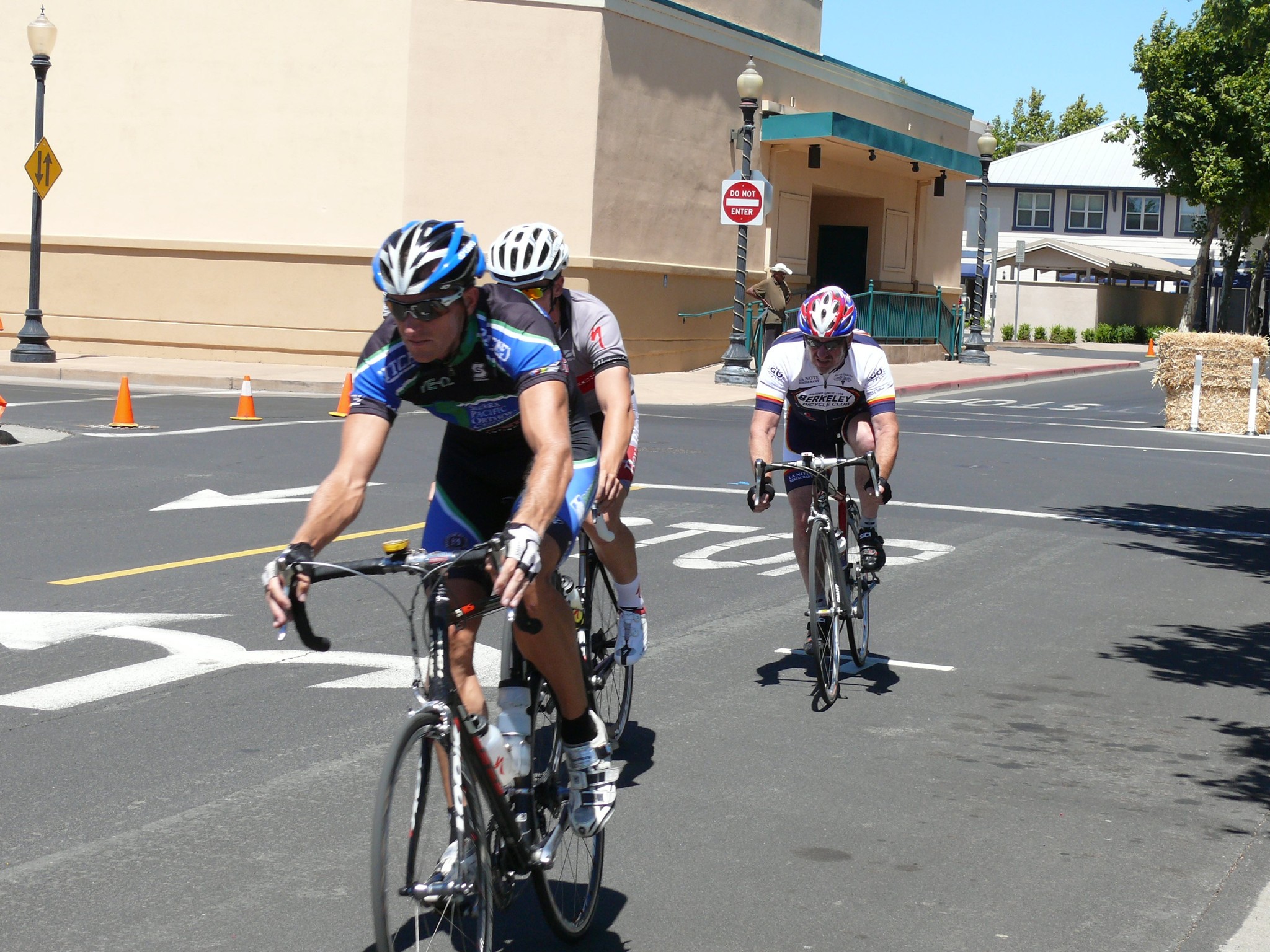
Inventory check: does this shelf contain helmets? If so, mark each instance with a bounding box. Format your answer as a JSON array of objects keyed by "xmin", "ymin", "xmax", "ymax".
[
  {"xmin": 372, "ymin": 219, "xmax": 486, "ymax": 295},
  {"xmin": 486, "ymin": 223, "xmax": 569, "ymax": 286},
  {"xmin": 797, "ymin": 286, "xmax": 858, "ymax": 343}
]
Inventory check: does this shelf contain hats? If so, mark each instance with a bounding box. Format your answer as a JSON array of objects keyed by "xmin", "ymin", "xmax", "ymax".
[{"xmin": 769, "ymin": 263, "xmax": 792, "ymax": 275}]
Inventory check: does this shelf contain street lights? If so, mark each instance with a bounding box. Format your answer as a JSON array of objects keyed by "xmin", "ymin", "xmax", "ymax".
[
  {"xmin": 9, "ymin": 4, "xmax": 58, "ymax": 363},
  {"xmin": 958, "ymin": 120, "xmax": 998, "ymax": 365},
  {"xmin": 714, "ymin": 54, "xmax": 765, "ymax": 389}
]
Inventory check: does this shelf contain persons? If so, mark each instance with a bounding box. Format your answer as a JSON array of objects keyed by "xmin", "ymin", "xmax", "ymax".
[
  {"xmin": 746, "ymin": 264, "xmax": 792, "ymax": 365},
  {"xmin": 484, "ymin": 224, "xmax": 650, "ymax": 665},
  {"xmin": 1258, "ymin": 307, "xmax": 1263, "ymax": 329},
  {"xmin": 750, "ymin": 286, "xmax": 898, "ymax": 656},
  {"xmin": 258, "ymin": 219, "xmax": 621, "ymax": 911}
]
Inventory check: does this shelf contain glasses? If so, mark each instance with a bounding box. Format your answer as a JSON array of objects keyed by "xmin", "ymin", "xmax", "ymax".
[
  {"xmin": 498, "ymin": 281, "xmax": 551, "ymax": 301},
  {"xmin": 383, "ymin": 287, "xmax": 466, "ymax": 323},
  {"xmin": 804, "ymin": 336, "xmax": 845, "ymax": 350}
]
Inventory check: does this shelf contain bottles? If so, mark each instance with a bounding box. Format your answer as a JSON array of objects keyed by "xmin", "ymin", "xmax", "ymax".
[
  {"xmin": 468, "ymin": 714, "xmax": 516, "ymax": 786},
  {"xmin": 834, "ymin": 527, "xmax": 848, "ymax": 569},
  {"xmin": 497, "ymin": 667, "xmax": 532, "ymax": 777},
  {"xmin": 559, "ymin": 575, "xmax": 584, "ymax": 624}
]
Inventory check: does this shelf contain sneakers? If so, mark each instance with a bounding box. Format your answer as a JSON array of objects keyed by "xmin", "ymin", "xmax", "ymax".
[
  {"xmin": 421, "ymin": 838, "xmax": 479, "ymax": 903},
  {"xmin": 614, "ymin": 605, "xmax": 648, "ymax": 666},
  {"xmin": 561, "ymin": 709, "xmax": 620, "ymax": 837},
  {"xmin": 857, "ymin": 527, "xmax": 886, "ymax": 572},
  {"xmin": 803, "ymin": 616, "xmax": 833, "ymax": 656}
]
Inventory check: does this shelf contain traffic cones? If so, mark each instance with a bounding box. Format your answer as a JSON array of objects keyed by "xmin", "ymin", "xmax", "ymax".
[
  {"xmin": 229, "ymin": 374, "xmax": 263, "ymax": 421},
  {"xmin": 108, "ymin": 376, "xmax": 139, "ymax": 428},
  {"xmin": 1145, "ymin": 338, "xmax": 1157, "ymax": 358},
  {"xmin": 327, "ymin": 373, "xmax": 353, "ymax": 418}
]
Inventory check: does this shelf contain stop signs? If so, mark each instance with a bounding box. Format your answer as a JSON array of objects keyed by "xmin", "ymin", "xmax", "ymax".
[{"xmin": 718, "ymin": 179, "xmax": 765, "ymax": 226}]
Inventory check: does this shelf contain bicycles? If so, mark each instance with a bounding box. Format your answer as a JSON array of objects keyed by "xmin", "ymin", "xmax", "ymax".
[
  {"xmin": 752, "ymin": 449, "xmax": 880, "ymax": 703},
  {"xmin": 281, "ymin": 519, "xmax": 635, "ymax": 951}
]
